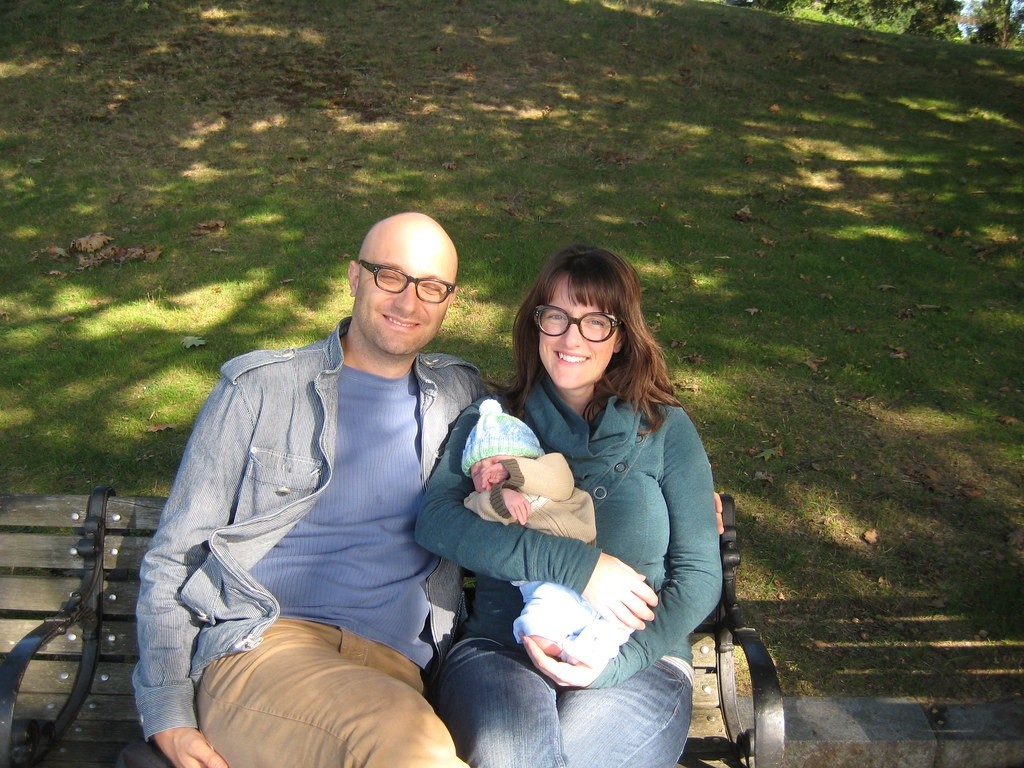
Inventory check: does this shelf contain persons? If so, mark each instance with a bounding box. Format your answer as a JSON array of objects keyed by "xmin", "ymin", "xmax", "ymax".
[
  {"xmin": 416, "ymin": 242, "xmax": 722, "ymax": 768},
  {"xmin": 129, "ymin": 210, "xmax": 721, "ymax": 767},
  {"xmin": 460, "ymin": 399, "xmax": 628, "ymax": 670}
]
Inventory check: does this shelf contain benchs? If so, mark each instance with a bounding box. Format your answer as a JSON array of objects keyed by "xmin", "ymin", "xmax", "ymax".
[{"xmin": 0, "ymin": 488, "xmax": 786, "ymax": 768}]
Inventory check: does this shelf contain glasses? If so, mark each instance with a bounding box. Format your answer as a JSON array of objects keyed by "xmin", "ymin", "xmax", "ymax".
[
  {"xmin": 533, "ymin": 305, "xmax": 623, "ymax": 343},
  {"xmin": 357, "ymin": 259, "xmax": 455, "ymax": 303}
]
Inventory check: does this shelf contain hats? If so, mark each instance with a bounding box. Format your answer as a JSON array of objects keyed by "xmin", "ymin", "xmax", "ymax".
[{"xmin": 461, "ymin": 400, "xmax": 545, "ymax": 478}]
